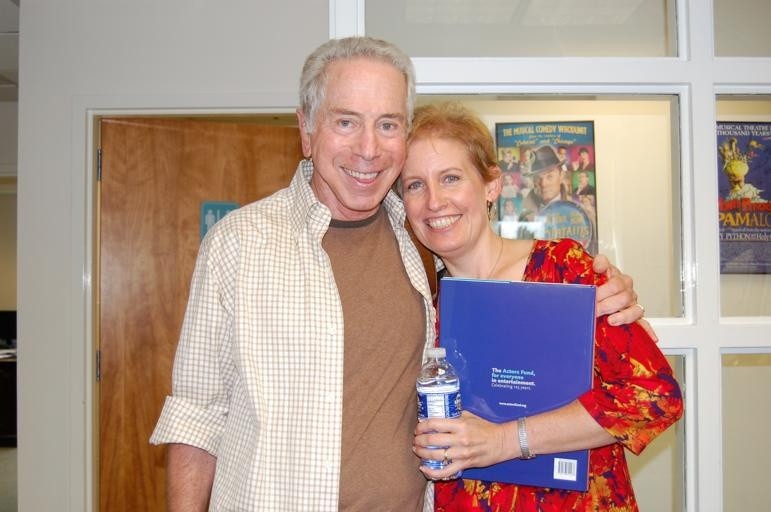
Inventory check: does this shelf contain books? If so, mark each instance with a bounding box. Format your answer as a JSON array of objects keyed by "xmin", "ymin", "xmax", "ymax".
[{"xmin": 436, "ymin": 275, "xmax": 597, "ymax": 492}]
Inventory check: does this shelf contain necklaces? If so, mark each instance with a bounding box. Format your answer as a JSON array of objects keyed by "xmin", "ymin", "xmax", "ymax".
[{"xmin": 487, "ymin": 236, "xmax": 503, "ymax": 280}]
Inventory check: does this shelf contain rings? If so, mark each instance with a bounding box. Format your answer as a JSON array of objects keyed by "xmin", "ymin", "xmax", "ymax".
[
  {"xmin": 638, "ymin": 304, "xmax": 645, "ymax": 313},
  {"xmin": 444, "ymin": 449, "xmax": 447, "ymax": 460}
]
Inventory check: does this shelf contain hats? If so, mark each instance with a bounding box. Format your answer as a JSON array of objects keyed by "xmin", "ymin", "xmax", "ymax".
[{"xmin": 524, "ymin": 147, "xmax": 566, "ymax": 177}]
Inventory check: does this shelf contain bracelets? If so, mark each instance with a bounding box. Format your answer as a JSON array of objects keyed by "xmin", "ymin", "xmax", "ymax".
[{"xmin": 517, "ymin": 414, "xmax": 535, "ymax": 460}]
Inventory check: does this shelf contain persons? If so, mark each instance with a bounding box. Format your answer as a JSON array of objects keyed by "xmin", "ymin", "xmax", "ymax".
[
  {"xmin": 491, "ymin": 145, "xmax": 597, "ymax": 255},
  {"xmin": 393, "ymin": 100, "xmax": 684, "ymax": 510},
  {"xmin": 147, "ymin": 36, "xmax": 658, "ymax": 511}
]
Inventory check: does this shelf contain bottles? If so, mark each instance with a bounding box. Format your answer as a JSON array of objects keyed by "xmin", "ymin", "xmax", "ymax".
[{"xmin": 415, "ymin": 347, "xmax": 465, "ymax": 481}]
